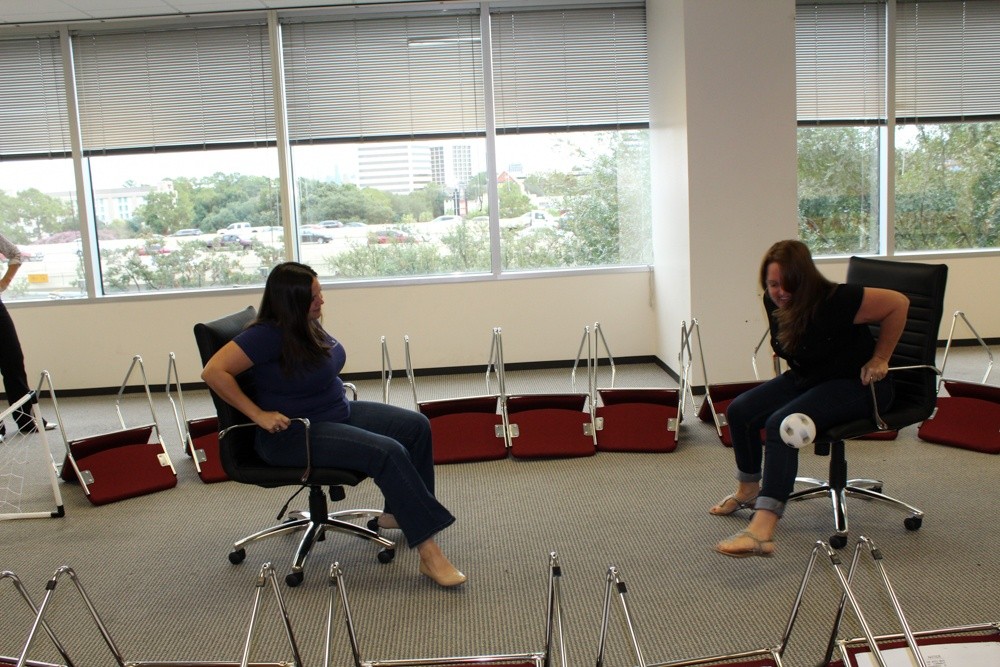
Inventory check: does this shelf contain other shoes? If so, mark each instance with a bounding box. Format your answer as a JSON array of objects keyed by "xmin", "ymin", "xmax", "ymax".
[
  {"xmin": 419, "ymin": 559, "xmax": 467, "ymax": 586},
  {"xmin": 44, "ymin": 422, "xmax": 58, "ymax": 430},
  {"xmin": 0, "ymin": 433, "xmax": 4, "ymax": 443},
  {"xmin": 377, "ymin": 511, "xmax": 401, "ymax": 528}
]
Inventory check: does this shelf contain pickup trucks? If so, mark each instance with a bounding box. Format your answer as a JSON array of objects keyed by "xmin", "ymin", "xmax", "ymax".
[{"xmin": 216, "ymin": 222, "xmax": 265, "ymax": 236}]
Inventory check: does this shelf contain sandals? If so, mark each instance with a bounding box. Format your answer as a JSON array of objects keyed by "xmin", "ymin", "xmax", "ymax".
[
  {"xmin": 714, "ymin": 530, "xmax": 776, "ymax": 558},
  {"xmin": 709, "ymin": 493, "xmax": 760, "ymax": 515}
]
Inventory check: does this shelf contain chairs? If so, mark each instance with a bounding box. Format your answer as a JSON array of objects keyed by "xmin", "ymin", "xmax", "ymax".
[{"xmin": 0, "ymin": 256, "xmax": 1000, "ymax": 667}]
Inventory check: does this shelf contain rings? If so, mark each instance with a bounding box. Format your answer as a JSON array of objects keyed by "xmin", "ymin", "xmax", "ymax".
[{"xmin": 275, "ymin": 427, "xmax": 281, "ymax": 432}]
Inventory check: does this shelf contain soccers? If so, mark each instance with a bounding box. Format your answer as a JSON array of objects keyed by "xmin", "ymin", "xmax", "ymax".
[{"xmin": 778, "ymin": 412, "xmax": 817, "ymax": 449}]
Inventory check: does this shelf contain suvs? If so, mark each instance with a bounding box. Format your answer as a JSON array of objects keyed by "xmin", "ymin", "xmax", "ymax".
[
  {"xmin": 168, "ymin": 228, "xmax": 203, "ymax": 236},
  {"xmin": 207, "ymin": 235, "xmax": 253, "ymax": 251}
]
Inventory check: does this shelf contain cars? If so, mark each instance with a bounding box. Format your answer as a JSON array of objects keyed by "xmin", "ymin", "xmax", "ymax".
[
  {"xmin": 367, "ymin": 229, "xmax": 421, "ymax": 243},
  {"xmin": 432, "ymin": 210, "xmax": 575, "ymax": 231},
  {"xmin": 320, "ymin": 219, "xmax": 343, "ymax": 228},
  {"xmin": 301, "ymin": 230, "xmax": 333, "ymax": 244},
  {"xmin": 0, "ymin": 251, "xmax": 43, "ymax": 263},
  {"xmin": 133, "ymin": 248, "xmax": 171, "ymax": 256}
]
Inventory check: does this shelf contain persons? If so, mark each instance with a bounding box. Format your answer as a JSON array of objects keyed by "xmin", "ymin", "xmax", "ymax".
[
  {"xmin": 0, "ymin": 234, "xmax": 58, "ymax": 443},
  {"xmin": 707, "ymin": 240, "xmax": 911, "ymax": 559},
  {"xmin": 200, "ymin": 261, "xmax": 467, "ymax": 588}
]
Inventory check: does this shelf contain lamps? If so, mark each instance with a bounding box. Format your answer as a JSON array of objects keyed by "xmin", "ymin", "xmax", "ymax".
[{"xmin": 407, "ymin": 37, "xmax": 481, "ymax": 47}]
